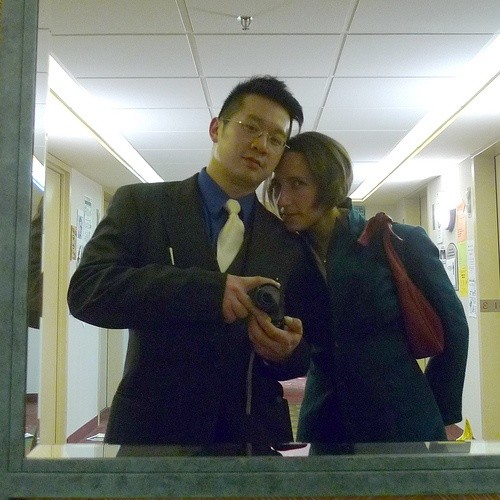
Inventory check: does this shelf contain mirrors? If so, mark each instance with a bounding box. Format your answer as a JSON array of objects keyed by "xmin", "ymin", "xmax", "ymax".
[{"xmin": 7, "ymin": 1, "xmax": 500, "ymax": 474}]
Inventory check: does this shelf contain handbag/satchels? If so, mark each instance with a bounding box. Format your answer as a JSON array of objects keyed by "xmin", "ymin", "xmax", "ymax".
[{"xmin": 359, "ymin": 211, "xmax": 444, "ymax": 359}]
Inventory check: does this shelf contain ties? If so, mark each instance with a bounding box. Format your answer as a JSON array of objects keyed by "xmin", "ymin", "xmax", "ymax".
[{"xmin": 216, "ymin": 201, "xmax": 245, "ymax": 275}]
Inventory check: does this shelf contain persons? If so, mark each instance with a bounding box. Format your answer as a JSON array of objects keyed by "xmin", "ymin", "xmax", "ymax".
[
  {"xmin": 67, "ymin": 75, "xmax": 330, "ymax": 443},
  {"xmin": 262, "ymin": 131, "xmax": 469, "ymax": 442}
]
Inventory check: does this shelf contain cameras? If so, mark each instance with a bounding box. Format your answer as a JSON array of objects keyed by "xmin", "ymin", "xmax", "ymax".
[{"xmin": 257, "ymin": 275, "xmax": 288, "ymax": 327}]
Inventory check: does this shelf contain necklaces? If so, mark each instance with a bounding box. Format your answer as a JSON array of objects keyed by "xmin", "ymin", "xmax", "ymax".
[{"xmin": 310, "ymin": 236, "xmax": 327, "ymax": 263}]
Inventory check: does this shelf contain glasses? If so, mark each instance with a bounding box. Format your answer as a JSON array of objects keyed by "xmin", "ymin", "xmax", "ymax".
[{"xmin": 222, "ymin": 118, "xmax": 290, "ymax": 151}]
